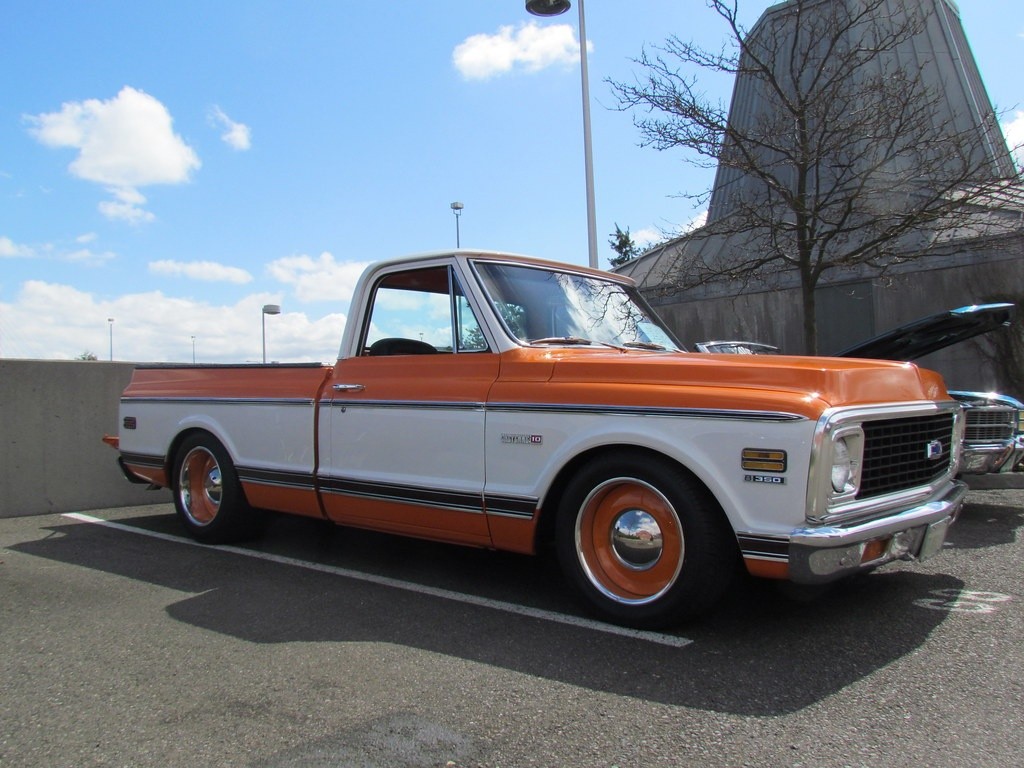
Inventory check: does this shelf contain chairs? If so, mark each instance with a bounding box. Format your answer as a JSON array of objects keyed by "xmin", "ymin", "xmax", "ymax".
[{"xmin": 369, "ymin": 337, "xmax": 438, "ymax": 355}]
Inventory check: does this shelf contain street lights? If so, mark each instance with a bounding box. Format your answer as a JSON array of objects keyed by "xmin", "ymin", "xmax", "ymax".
[
  {"xmin": 261, "ymin": 304, "xmax": 282, "ymax": 364},
  {"xmin": 524, "ymin": 0, "xmax": 599, "ymax": 270},
  {"xmin": 108, "ymin": 318, "xmax": 115, "ymax": 361},
  {"xmin": 191, "ymin": 335, "xmax": 196, "ymax": 363},
  {"xmin": 451, "ymin": 202, "xmax": 464, "ymax": 349}
]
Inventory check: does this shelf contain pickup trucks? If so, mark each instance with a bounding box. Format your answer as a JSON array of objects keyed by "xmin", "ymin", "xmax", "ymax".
[{"xmin": 102, "ymin": 251, "xmax": 973, "ymax": 631}]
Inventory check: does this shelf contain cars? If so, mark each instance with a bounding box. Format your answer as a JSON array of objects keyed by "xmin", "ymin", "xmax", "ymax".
[{"xmin": 692, "ymin": 300, "xmax": 1024, "ymax": 490}]
